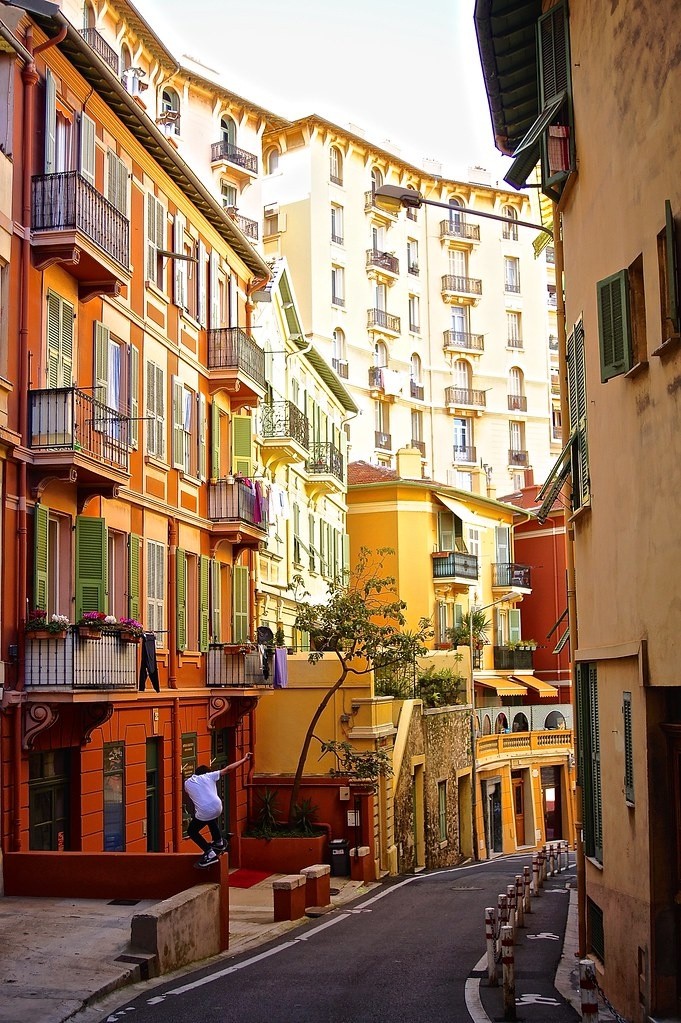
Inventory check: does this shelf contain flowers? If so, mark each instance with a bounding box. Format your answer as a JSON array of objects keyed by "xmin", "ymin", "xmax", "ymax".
[
  {"xmin": 77, "ymin": 611, "xmax": 115, "ymax": 630},
  {"xmin": 118, "ymin": 617, "xmax": 142, "ymax": 637},
  {"xmin": 22, "ymin": 609, "xmax": 71, "ymax": 634}
]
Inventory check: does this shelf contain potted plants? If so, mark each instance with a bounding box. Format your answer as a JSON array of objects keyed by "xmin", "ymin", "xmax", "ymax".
[{"xmin": 517, "ymin": 639, "xmax": 537, "ymax": 650}]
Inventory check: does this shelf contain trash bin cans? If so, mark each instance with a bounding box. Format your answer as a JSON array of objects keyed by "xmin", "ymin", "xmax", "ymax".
[{"xmin": 328, "ymin": 838, "xmax": 350, "ymax": 877}]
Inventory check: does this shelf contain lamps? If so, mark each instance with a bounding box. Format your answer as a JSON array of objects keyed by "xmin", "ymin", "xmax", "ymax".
[{"xmin": 373, "ymin": 184, "xmax": 554, "ymax": 241}]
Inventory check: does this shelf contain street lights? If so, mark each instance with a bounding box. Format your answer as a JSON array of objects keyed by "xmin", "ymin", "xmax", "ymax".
[{"xmin": 469, "ymin": 592, "xmax": 523, "ymax": 714}]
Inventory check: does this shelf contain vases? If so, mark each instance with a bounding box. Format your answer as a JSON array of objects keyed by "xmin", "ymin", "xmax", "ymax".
[
  {"xmin": 120, "ymin": 630, "xmax": 141, "ymax": 643},
  {"xmin": 26, "ymin": 628, "xmax": 67, "ymax": 639},
  {"xmin": 78, "ymin": 627, "xmax": 102, "ymax": 639}
]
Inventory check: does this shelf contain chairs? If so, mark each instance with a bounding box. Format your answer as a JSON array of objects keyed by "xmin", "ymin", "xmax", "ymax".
[{"xmin": 511, "ymin": 571, "xmax": 525, "ymax": 586}]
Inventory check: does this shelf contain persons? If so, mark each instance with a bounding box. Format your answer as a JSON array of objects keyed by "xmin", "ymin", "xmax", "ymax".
[{"xmin": 185, "ymin": 752, "xmax": 253, "ymax": 866}]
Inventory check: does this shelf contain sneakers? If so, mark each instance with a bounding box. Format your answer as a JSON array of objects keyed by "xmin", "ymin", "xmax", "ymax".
[
  {"xmin": 209, "ymin": 841, "xmax": 226, "ymax": 850},
  {"xmin": 199, "ymin": 854, "xmax": 219, "ymax": 866}
]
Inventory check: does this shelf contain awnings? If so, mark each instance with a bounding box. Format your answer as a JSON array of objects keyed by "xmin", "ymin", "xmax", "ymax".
[
  {"xmin": 474, "ymin": 679, "xmax": 527, "ymax": 696},
  {"xmin": 434, "ymin": 492, "xmax": 487, "ymax": 528},
  {"xmin": 512, "ymin": 675, "xmax": 558, "ymax": 697}
]
